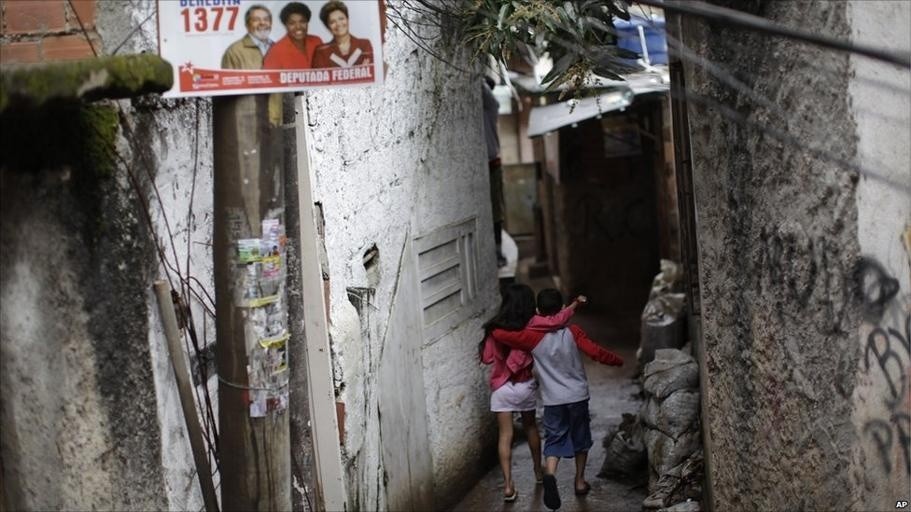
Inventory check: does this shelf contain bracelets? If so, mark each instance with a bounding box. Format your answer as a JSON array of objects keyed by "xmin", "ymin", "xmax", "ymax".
[{"xmin": 573, "ymin": 296, "xmax": 583, "ymax": 306}]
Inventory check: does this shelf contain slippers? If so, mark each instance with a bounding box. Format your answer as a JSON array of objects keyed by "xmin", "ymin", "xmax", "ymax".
[
  {"xmin": 503, "ymin": 480, "xmax": 517, "ymax": 500},
  {"xmin": 536, "ymin": 464, "xmax": 543, "ymax": 483}
]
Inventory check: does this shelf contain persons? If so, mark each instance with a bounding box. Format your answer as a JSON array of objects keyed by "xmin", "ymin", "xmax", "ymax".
[
  {"xmin": 490, "ymin": 287, "xmax": 625, "ymax": 509},
  {"xmin": 313, "ymin": 1, "xmax": 375, "ymax": 69},
  {"xmin": 222, "ymin": 5, "xmax": 276, "ymax": 71},
  {"xmin": 263, "ymin": 2, "xmax": 324, "ymax": 70},
  {"xmin": 479, "ymin": 281, "xmax": 589, "ymax": 502}
]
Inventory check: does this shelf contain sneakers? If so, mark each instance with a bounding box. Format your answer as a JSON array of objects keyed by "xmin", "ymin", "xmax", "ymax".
[
  {"xmin": 575, "ymin": 481, "xmax": 591, "ymax": 495},
  {"xmin": 543, "ymin": 474, "xmax": 561, "ymax": 509}
]
